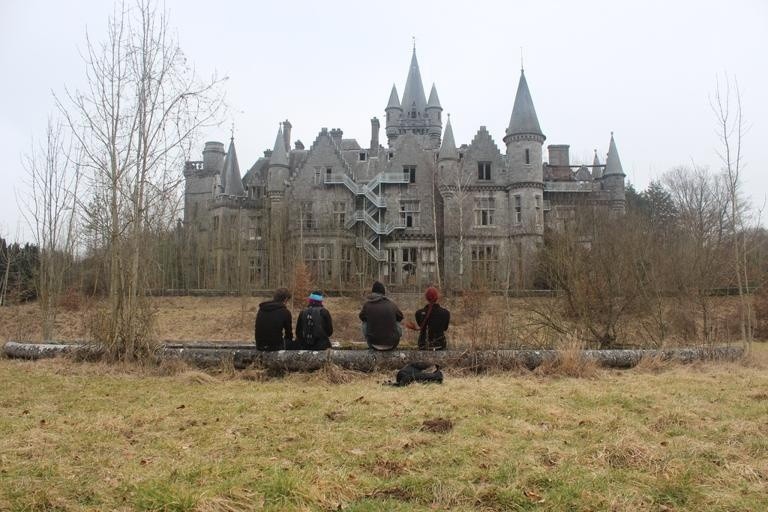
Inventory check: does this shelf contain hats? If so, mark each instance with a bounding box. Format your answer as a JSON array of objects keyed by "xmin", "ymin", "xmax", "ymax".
[
  {"xmin": 306, "ymin": 292, "xmax": 324, "ymax": 304},
  {"xmin": 372, "ymin": 282, "xmax": 385, "ymax": 295}
]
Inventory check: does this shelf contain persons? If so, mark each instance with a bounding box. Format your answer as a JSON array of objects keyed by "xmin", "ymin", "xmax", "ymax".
[
  {"xmin": 358, "ymin": 281, "xmax": 404, "ymax": 351},
  {"xmin": 255, "ymin": 287, "xmax": 333, "ymax": 350},
  {"xmin": 404, "ymin": 287, "xmax": 450, "ymax": 351}
]
